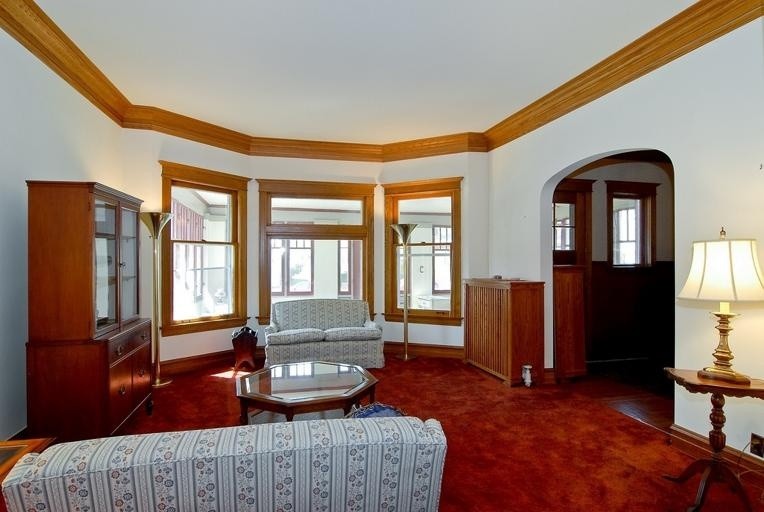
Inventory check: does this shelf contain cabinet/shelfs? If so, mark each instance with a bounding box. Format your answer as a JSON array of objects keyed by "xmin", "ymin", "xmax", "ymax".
[
  {"xmin": 22, "ymin": 179, "xmax": 146, "ymax": 341},
  {"xmin": 551, "ymin": 259, "xmax": 592, "ymax": 386},
  {"xmin": 460, "ymin": 274, "xmax": 546, "ymax": 390},
  {"xmin": 21, "ymin": 317, "xmax": 155, "ymax": 444}
]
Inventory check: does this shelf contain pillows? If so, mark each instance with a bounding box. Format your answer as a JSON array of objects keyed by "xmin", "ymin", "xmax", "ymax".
[{"xmin": 340, "ymin": 398, "xmax": 407, "ymax": 418}]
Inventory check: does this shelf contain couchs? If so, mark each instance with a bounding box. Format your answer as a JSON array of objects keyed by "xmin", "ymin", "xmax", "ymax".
[
  {"xmin": 259, "ymin": 298, "xmax": 388, "ymax": 376},
  {"xmin": 0, "ymin": 405, "xmax": 449, "ymax": 512}
]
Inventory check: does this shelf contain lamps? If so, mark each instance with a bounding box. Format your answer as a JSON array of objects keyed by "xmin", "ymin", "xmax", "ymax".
[
  {"xmin": 135, "ymin": 208, "xmax": 177, "ymax": 388},
  {"xmin": 675, "ymin": 223, "xmax": 763, "ymax": 385},
  {"xmin": 387, "ymin": 221, "xmax": 423, "ymax": 362}
]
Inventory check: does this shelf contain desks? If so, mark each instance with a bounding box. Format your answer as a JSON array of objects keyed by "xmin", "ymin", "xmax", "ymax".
[
  {"xmin": 658, "ymin": 362, "xmax": 764, "ymax": 512},
  {"xmin": 0, "ymin": 433, "xmax": 62, "ymax": 498}
]
